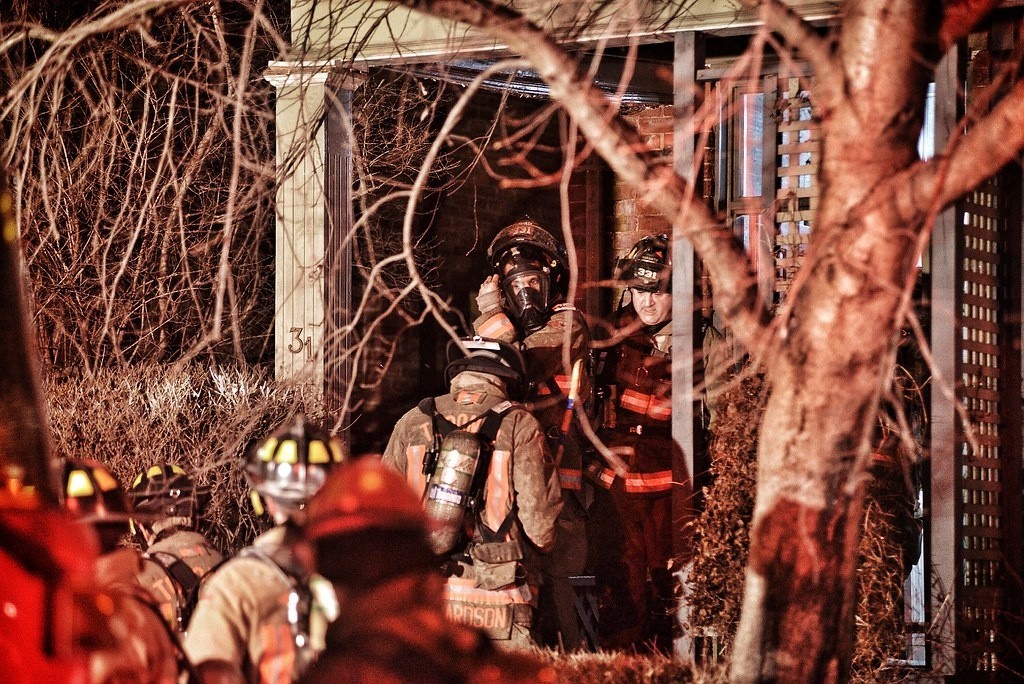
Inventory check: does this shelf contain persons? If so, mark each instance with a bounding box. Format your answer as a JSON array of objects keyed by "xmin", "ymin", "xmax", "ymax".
[
  {"xmin": 291, "ymin": 459, "xmax": 501, "ymax": 684},
  {"xmin": 54, "ymin": 424, "xmax": 348, "ymax": 684},
  {"xmin": 379, "ymin": 336, "xmax": 563, "ymax": 650},
  {"xmin": 592, "ymin": 235, "xmax": 729, "ymax": 656},
  {"xmin": 473, "ymin": 220, "xmax": 600, "ymax": 653}
]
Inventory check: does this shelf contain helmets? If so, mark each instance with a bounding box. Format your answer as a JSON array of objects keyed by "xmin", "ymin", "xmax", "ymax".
[
  {"xmin": 65, "ymin": 460, "xmax": 131, "ymax": 528},
  {"xmin": 446, "ymin": 337, "xmax": 528, "ymax": 403},
  {"xmin": 619, "ymin": 233, "xmax": 672, "ymax": 294},
  {"xmin": 242, "ymin": 413, "xmax": 346, "ymax": 507},
  {"xmin": 487, "ymin": 219, "xmax": 570, "ymax": 286},
  {"xmin": 132, "ymin": 463, "xmax": 190, "ymax": 503},
  {"xmin": 305, "ymin": 453, "xmax": 430, "ymax": 547}
]
